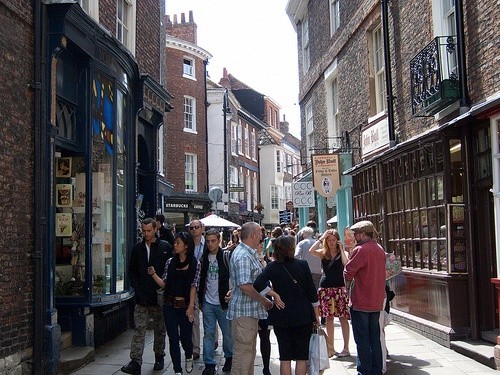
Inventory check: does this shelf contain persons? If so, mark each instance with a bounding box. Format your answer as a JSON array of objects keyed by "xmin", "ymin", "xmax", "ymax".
[
  {"xmin": 120, "ymin": 218, "xmax": 164, "ymax": 375},
  {"xmin": 155, "ymin": 214, "xmax": 175, "ymax": 250},
  {"xmin": 253, "ymin": 235, "xmax": 321, "ymax": 375},
  {"xmin": 186, "ymin": 227, "xmax": 232, "ymax": 375},
  {"xmin": 224, "ymin": 220, "xmax": 325, "ymax": 375},
  {"xmin": 147, "ymin": 232, "xmax": 194, "ymax": 375},
  {"xmin": 307, "ymin": 220, "xmax": 394, "ymax": 375},
  {"xmin": 225, "ymin": 221, "xmax": 273, "ymax": 375},
  {"xmin": 187, "ymin": 219, "xmax": 219, "ymax": 359}
]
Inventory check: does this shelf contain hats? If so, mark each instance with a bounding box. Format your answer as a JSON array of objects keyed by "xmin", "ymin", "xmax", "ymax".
[{"xmin": 349, "ymin": 220, "xmax": 375, "ymax": 233}]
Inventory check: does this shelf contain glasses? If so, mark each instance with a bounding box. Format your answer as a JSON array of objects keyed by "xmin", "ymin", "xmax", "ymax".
[
  {"xmin": 260, "ymin": 240, "xmax": 263, "ymax": 244},
  {"xmin": 353, "ymin": 232, "xmax": 363, "ymax": 237},
  {"xmin": 190, "ymin": 226, "xmax": 202, "ymax": 230}
]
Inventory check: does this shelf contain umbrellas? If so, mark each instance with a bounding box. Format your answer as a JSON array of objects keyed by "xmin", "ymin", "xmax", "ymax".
[{"xmin": 184, "ymin": 213, "xmax": 242, "ymax": 228}]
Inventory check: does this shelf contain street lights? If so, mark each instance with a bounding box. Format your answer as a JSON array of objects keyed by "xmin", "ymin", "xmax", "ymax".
[{"xmin": 221, "ymin": 87, "xmax": 234, "ymax": 220}]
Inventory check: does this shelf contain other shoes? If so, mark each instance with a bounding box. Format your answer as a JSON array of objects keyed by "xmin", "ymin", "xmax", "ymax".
[
  {"xmin": 121, "ymin": 360, "xmax": 141, "ymax": 375},
  {"xmin": 202, "ymin": 363, "xmax": 216, "ymax": 375},
  {"xmin": 185, "ymin": 354, "xmax": 194, "ymax": 373},
  {"xmin": 222, "ymin": 357, "xmax": 232, "ymax": 372},
  {"xmin": 153, "ymin": 357, "xmax": 164, "ymax": 370},
  {"xmin": 338, "ymin": 349, "xmax": 350, "ymax": 357},
  {"xmin": 172, "ymin": 359, "xmax": 183, "ymax": 375},
  {"xmin": 192, "ymin": 353, "xmax": 199, "ymax": 360},
  {"xmin": 214, "ymin": 341, "xmax": 218, "ymax": 350},
  {"xmin": 262, "ymin": 368, "xmax": 272, "ymax": 375}
]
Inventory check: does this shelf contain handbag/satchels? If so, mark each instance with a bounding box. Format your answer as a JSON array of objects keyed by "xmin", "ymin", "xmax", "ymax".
[
  {"xmin": 157, "ymin": 288, "xmax": 165, "ymax": 308},
  {"xmin": 309, "ymin": 324, "xmax": 335, "ymax": 375},
  {"xmin": 385, "ymin": 251, "xmax": 402, "ymax": 281}
]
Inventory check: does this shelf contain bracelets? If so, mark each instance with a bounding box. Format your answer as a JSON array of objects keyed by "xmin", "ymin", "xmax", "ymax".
[
  {"xmin": 150, "ymin": 271, "xmax": 156, "ymax": 276},
  {"xmin": 318, "ymin": 238, "xmax": 323, "ymax": 244}
]
